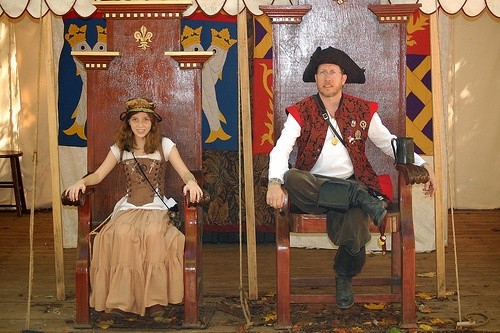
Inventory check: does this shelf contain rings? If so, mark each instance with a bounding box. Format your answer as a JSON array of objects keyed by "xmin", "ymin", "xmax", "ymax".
[{"xmin": 270, "ymin": 201, "xmax": 272, "ymax": 204}]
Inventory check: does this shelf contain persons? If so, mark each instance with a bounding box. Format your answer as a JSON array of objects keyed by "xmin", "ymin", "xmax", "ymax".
[
  {"xmin": 65, "ymin": 96, "xmax": 203, "ymax": 322},
  {"xmin": 266, "ymin": 45, "xmax": 436, "ymax": 308}
]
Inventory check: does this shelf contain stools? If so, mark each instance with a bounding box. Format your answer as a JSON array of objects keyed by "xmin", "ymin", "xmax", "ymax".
[{"xmin": 0, "ymin": 150, "xmax": 27, "ymax": 215}]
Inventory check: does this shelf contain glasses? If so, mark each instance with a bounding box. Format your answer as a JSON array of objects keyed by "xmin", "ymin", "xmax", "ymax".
[{"xmin": 317, "ymin": 71, "xmax": 342, "ymax": 76}]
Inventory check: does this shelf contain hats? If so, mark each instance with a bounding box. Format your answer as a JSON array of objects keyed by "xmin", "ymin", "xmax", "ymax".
[
  {"xmin": 303, "ymin": 46, "xmax": 365, "ymax": 84},
  {"xmin": 120, "ymin": 98, "xmax": 162, "ymax": 122}
]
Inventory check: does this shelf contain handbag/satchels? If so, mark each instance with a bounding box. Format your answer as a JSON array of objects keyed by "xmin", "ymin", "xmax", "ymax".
[
  {"xmin": 167, "ymin": 205, "xmax": 183, "ymax": 229},
  {"xmin": 370, "ymin": 175, "xmax": 393, "ymax": 209}
]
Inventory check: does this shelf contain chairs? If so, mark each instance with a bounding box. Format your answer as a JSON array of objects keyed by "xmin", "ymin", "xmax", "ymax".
[
  {"xmin": 259, "ymin": 1, "xmax": 428, "ymax": 325},
  {"xmin": 68, "ymin": 1, "xmax": 216, "ymax": 329}
]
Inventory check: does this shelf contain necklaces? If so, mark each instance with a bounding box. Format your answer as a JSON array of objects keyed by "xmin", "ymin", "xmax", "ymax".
[{"xmin": 329, "ymin": 124, "xmax": 338, "ymax": 145}]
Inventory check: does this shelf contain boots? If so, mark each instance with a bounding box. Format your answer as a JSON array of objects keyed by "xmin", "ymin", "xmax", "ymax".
[
  {"xmin": 312, "ymin": 174, "xmax": 387, "ymax": 228},
  {"xmin": 332, "ymin": 245, "xmax": 366, "ymax": 308}
]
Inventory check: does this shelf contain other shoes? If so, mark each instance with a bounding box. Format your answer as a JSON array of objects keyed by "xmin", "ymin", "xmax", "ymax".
[
  {"xmin": 149, "ymin": 304, "xmax": 176, "ymax": 323},
  {"xmin": 99, "ymin": 308, "xmax": 137, "ymax": 322}
]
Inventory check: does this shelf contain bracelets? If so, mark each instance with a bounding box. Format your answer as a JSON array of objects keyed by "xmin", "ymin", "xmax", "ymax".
[{"xmin": 269, "ymin": 178, "xmax": 281, "ymax": 184}]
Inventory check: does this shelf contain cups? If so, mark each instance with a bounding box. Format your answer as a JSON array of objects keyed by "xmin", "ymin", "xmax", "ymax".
[{"xmin": 391, "ymin": 137, "xmax": 415, "ymax": 165}]
